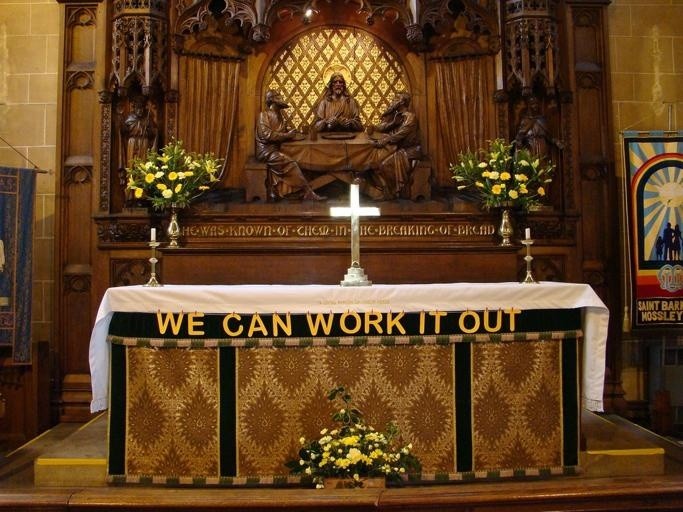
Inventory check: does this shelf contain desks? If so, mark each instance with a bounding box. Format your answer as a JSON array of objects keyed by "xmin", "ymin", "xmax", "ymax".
[{"xmin": 88, "ymin": 284, "xmax": 608, "ymax": 482}]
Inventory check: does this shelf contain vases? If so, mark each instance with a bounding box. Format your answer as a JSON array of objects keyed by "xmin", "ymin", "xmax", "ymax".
[
  {"xmin": 498, "ymin": 210, "xmax": 514, "ymax": 247},
  {"xmin": 166, "ymin": 214, "xmax": 181, "ymax": 248},
  {"xmin": 323, "ymin": 477, "xmax": 385, "ymax": 488}
]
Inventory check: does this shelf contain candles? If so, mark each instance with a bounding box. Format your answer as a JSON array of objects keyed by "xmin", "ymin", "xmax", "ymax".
[
  {"xmin": 350, "ymin": 184, "xmax": 359, "ymax": 208},
  {"xmin": 151, "ymin": 228, "xmax": 156, "ymax": 242},
  {"xmin": 525, "ymin": 228, "xmax": 530, "ymax": 240}
]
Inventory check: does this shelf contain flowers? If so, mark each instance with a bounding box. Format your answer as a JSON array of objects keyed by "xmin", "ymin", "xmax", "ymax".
[
  {"xmin": 285, "ymin": 387, "xmax": 419, "ymax": 489},
  {"xmin": 448, "ymin": 138, "xmax": 556, "ymax": 211},
  {"xmin": 122, "ymin": 136, "xmax": 225, "ymax": 214}
]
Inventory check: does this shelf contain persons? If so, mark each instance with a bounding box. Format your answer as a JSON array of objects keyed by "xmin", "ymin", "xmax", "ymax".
[
  {"xmin": 256, "ymin": 90, "xmax": 327, "ymax": 201},
  {"xmin": 117, "ymin": 99, "xmax": 159, "ymax": 207},
  {"xmin": 514, "ymin": 97, "xmax": 567, "ymax": 201},
  {"xmin": 655, "ymin": 223, "xmax": 683, "ymax": 261},
  {"xmin": 374, "ymin": 92, "xmax": 423, "ymax": 201},
  {"xmin": 311, "ymin": 72, "xmax": 363, "ymax": 133}
]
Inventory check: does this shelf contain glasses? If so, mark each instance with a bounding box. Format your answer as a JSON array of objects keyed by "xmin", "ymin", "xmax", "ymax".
[{"xmin": 266, "ymin": 89, "xmax": 280, "ymax": 98}]
